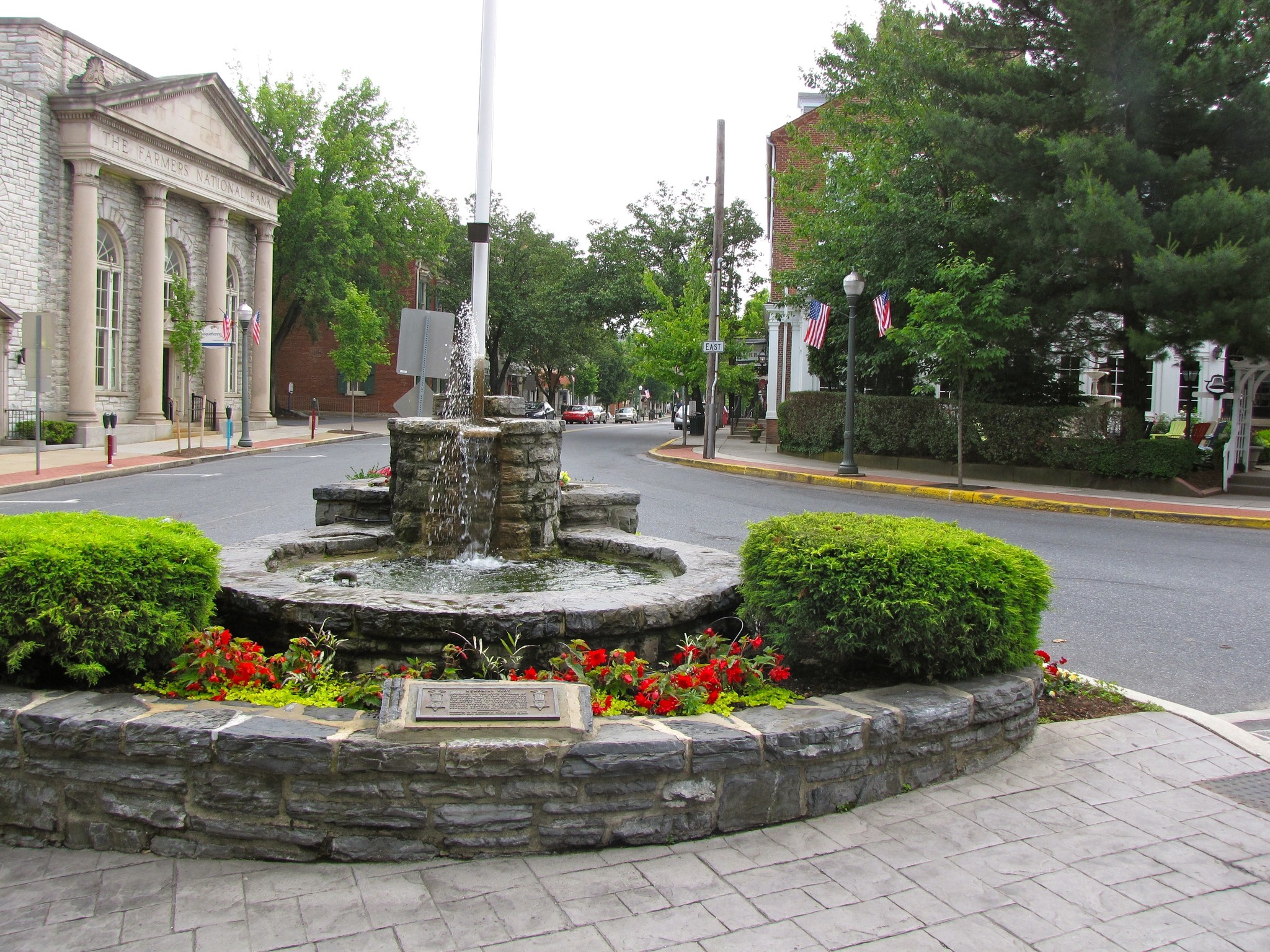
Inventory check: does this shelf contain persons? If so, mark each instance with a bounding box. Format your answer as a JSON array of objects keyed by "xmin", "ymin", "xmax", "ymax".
[
  {"xmin": 723, "ymin": 406, "xmax": 728, "ymax": 428},
  {"xmin": 676, "ymin": 400, "xmax": 683, "ymax": 412}
]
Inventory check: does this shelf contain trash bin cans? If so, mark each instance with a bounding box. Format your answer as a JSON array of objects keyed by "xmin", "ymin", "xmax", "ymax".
[{"xmin": 688, "ymin": 411, "xmax": 705, "ymax": 436}]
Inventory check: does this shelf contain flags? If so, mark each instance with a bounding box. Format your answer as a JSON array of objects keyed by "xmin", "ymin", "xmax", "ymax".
[
  {"xmin": 222, "ymin": 313, "xmax": 233, "ymax": 343},
  {"xmin": 872, "ymin": 288, "xmax": 893, "ymax": 338},
  {"xmin": 803, "ymin": 299, "xmax": 831, "ymax": 351},
  {"xmin": 251, "ymin": 310, "xmax": 261, "ymax": 345}
]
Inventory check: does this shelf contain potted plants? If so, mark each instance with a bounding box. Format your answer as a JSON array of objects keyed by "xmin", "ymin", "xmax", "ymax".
[{"xmin": 744, "ymin": 399, "xmax": 764, "ymax": 443}]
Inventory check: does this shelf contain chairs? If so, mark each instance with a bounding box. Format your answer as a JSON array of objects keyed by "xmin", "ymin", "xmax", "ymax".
[{"xmin": 1147, "ymin": 420, "xmax": 1229, "ymax": 451}]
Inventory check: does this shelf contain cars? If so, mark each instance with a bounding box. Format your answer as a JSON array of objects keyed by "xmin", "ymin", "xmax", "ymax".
[
  {"xmin": 615, "ymin": 406, "xmax": 637, "ymax": 424},
  {"xmin": 524, "ymin": 401, "xmax": 557, "ymax": 421},
  {"xmin": 672, "ymin": 404, "xmax": 730, "ymax": 432},
  {"xmin": 562, "ymin": 404, "xmax": 595, "ymax": 424},
  {"xmin": 588, "ymin": 405, "xmax": 608, "ymax": 423}
]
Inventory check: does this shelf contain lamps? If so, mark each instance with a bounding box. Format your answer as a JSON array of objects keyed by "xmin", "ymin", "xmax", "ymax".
[{"xmin": 757, "ymin": 345, "xmax": 768, "ymax": 368}]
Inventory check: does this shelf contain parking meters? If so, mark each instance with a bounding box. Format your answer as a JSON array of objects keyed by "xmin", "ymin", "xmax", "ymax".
[
  {"xmin": 308, "ymin": 397, "xmax": 319, "ymax": 439},
  {"xmin": 225, "ymin": 406, "xmax": 234, "ymax": 451},
  {"xmin": 103, "ymin": 411, "xmax": 120, "ymax": 467}
]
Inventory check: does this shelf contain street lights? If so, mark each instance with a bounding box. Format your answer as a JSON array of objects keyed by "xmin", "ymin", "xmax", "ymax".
[
  {"xmin": 834, "ymin": 265, "xmax": 869, "ymax": 477},
  {"xmin": 235, "ymin": 299, "xmax": 255, "ymax": 448}
]
